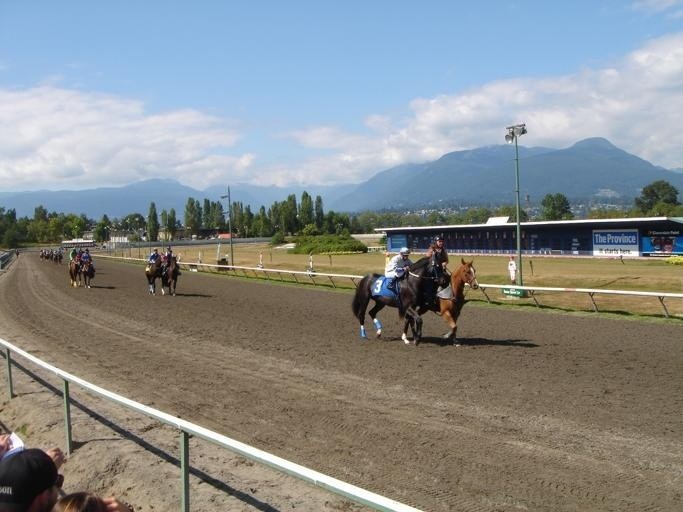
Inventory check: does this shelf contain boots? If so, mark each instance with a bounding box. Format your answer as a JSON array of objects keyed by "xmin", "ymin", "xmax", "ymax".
[{"xmin": 386, "ymin": 276, "xmax": 398, "ymax": 289}]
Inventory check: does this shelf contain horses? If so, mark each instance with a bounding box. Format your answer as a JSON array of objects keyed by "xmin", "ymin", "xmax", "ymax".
[
  {"xmin": 68, "ymin": 254, "xmax": 94, "ymax": 289},
  {"xmin": 351, "ymin": 246, "xmax": 449, "ymax": 345},
  {"xmin": 408, "ymin": 257, "xmax": 479, "ymax": 347},
  {"xmin": 145, "ymin": 254, "xmax": 179, "ymax": 296},
  {"xmin": 40, "ymin": 251, "xmax": 63, "ymax": 265},
  {"xmin": 16, "ymin": 251, "xmax": 19, "ymax": 258}
]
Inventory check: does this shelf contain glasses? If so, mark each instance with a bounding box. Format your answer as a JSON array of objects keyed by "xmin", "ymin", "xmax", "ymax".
[{"xmin": 50, "ymin": 474, "xmax": 63, "ymax": 488}]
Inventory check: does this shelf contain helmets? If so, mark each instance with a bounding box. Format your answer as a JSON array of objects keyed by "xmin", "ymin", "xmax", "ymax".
[
  {"xmin": 433, "ymin": 235, "xmax": 444, "ymax": 242},
  {"xmin": 399, "ymin": 247, "xmax": 410, "ymax": 256}
]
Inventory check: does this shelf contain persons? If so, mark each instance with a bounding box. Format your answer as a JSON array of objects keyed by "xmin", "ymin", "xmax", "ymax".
[
  {"xmin": 40, "ymin": 247, "xmax": 95, "ymax": 272},
  {"xmin": 425, "ymin": 235, "xmax": 449, "ymax": 270},
  {"xmin": 146, "ymin": 247, "xmax": 181, "ymax": 275},
  {"xmin": 385, "ymin": 247, "xmax": 415, "ymax": 289},
  {"xmin": 508, "ymin": 255, "xmax": 517, "ymax": 283},
  {"xmin": 0, "ymin": 434, "xmax": 136, "ymax": 512}
]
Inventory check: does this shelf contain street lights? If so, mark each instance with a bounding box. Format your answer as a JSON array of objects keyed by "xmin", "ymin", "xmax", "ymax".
[
  {"xmin": 504, "ymin": 123, "xmax": 527, "ymax": 285},
  {"xmin": 222, "ymin": 187, "xmax": 234, "ymax": 265},
  {"xmin": 104, "ymin": 212, "xmax": 164, "ymax": 258}
]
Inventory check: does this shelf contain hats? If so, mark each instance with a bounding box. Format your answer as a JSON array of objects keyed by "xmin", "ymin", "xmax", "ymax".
[{"xmin": 0, "ymin": 448, "xmax": 57, "ymax": 512}]
